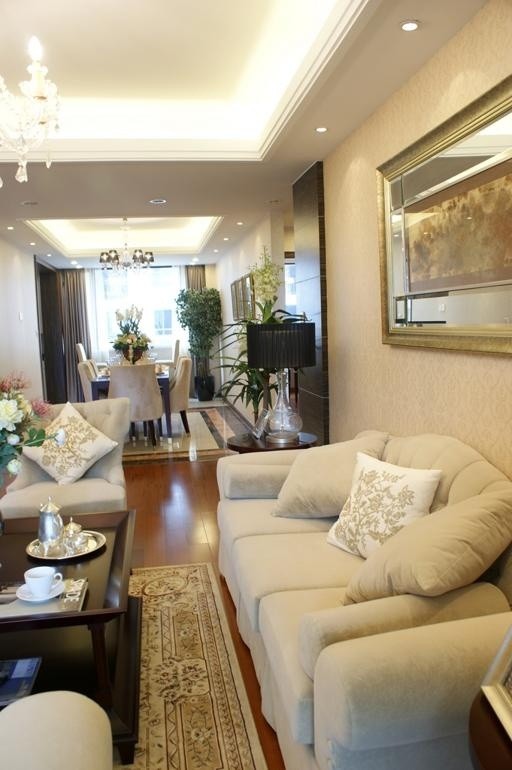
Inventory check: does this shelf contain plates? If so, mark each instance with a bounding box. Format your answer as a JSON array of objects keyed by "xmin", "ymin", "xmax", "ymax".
[{"xmin": 15, "ymin": 583, "xmax": 65, "ymax": 603}]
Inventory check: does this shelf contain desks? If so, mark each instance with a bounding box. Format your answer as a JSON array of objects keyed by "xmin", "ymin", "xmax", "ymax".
[
  {"xmin": 227, "ymin": 432, "xmax": 318, "ymax": 453},
  {"xmin": 462, "ymin": 693, "xmax": 511, "ymax": 765}
]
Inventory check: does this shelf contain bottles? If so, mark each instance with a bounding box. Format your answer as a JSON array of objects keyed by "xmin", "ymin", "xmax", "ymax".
[
  {"xmin": 64, "ymin": 517, "xmax": 81, "ymax": 536},
  {"xmin": 38, "ymin": 496, "xmax": 63, "ymax": 541}
]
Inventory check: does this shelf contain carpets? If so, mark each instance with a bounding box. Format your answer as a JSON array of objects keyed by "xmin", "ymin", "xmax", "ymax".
[
  {"xmin": 119, "ymin": 562, "xmax": 264, "ymax": 769},
  {"xmin": 119, "ymin": 404, "xmax": 255, "ymax": 466}
]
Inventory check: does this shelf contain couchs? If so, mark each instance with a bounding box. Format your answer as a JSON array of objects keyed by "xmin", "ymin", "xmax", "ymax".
[{"xmin": 215, "ymin": 421, "xmax": 512, "ymax": 769}]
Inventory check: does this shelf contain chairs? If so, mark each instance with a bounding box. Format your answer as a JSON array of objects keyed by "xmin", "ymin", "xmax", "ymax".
[
  {"xmin": 0, "ymin": 685, "xmax": 121, "ymax": 769},
  {"xmin": 1, "ymin": 396, "xmax": 135, "ymax": 577},
  {"xmin": 72, "ymin": 339, "xmax": 193, "ymax": 448}
]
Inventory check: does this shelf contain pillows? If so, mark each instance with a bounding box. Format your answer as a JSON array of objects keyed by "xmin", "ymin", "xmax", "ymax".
[
  {"xmin": 345, "ymin": 487, "xmax": 510, "ymax": 611},
  {"xmin": 326, "ymin": 454, "xmax": 441, "ymax": 566},
  {"xmin": 23, "ymin": 402, "xmax": 122, "ymax": 484},
  {"xmin": 270, "ymin": 433, "xmax": 392, "ymax": 518}
]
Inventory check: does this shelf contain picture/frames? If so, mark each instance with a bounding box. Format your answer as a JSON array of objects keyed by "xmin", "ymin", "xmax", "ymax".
[
  {"xmin": 374, "ymin": 70, "xmax": 512, "ymax": 355},
  {"xmin": 229, "ymin": 273, "xmax": 258, "ymax": 322},
  {"xmin": 482, "ymin": 635, "xmax": 511, "ymax": 740}
]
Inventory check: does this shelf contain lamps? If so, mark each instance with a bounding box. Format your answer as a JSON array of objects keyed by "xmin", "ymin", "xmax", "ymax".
[
  {"xmin": 0, "ymin": 38, "xmax": 61, "ymax": 186},
  {"xmin": 241, "ymin": 317, "xmax": 317, "ymax": 442},
  {"xmin": 97, "ymin": 216, "xmax": 155, "ymax": 280}
]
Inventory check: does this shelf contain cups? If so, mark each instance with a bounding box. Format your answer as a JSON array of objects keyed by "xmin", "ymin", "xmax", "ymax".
[
  {"xmin": 63, "ymin": 537, "xmax": 92, "ymax": 555},
  {"xmin": 23, "ymin": 564, "xmax": 62, "ymax": 597}
]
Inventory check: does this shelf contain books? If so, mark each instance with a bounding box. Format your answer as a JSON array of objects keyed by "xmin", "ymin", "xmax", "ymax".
[
  {"xmin": 0, "ymin": 577, "xmax": 88, "ymax": 611},
  {"xmin": 0, "ymin": 656, "xmax": 42, "ymax": 707}
]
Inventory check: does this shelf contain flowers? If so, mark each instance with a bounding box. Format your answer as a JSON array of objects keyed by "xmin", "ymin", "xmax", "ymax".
[
  {"xmin": 0, "ymin": 369, "xmax": 66, "ymax": 487},
  {"xmin": 112, "ymin": 307, "xmax": 152, "ymax": 350}
]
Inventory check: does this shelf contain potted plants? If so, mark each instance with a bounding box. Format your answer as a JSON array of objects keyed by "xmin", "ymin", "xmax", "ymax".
[{"xmin": 172, "ymin": 284, "xmax": 225, "ymax": 402}]
